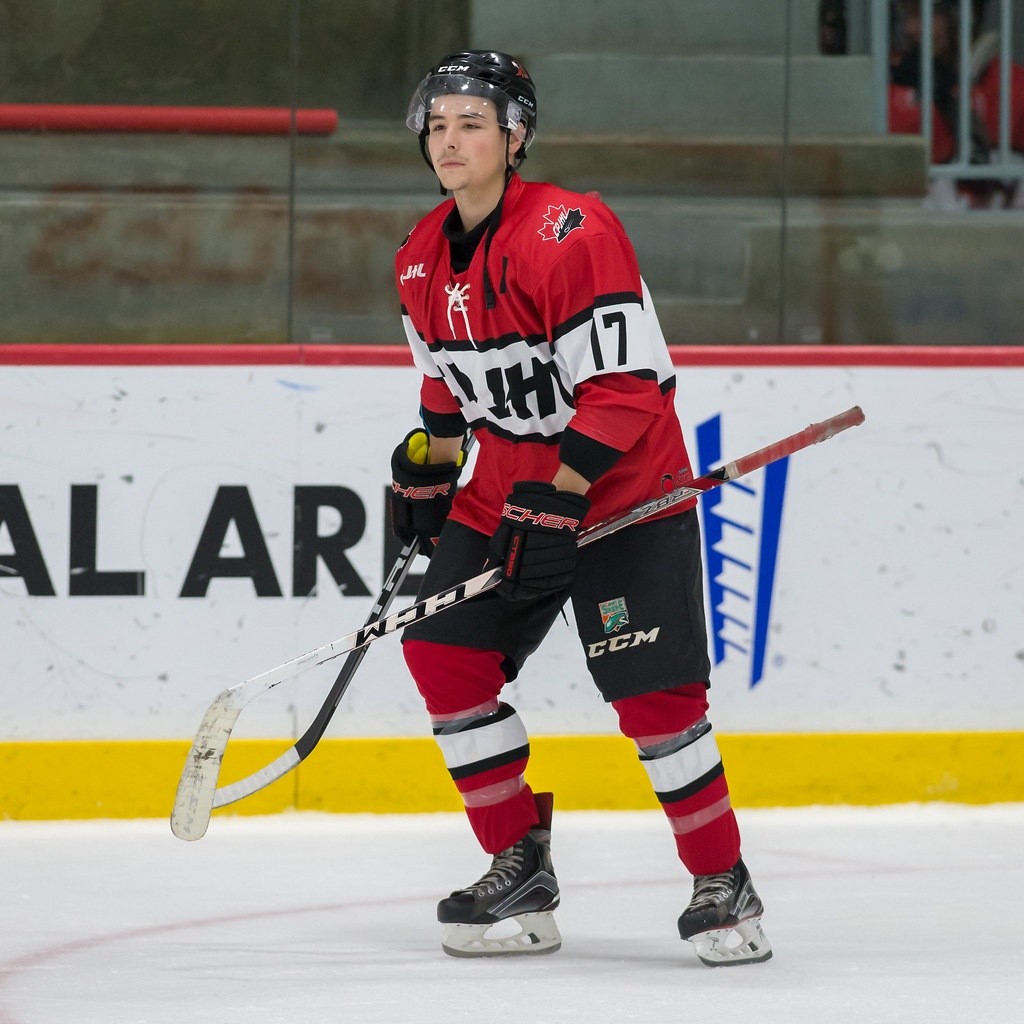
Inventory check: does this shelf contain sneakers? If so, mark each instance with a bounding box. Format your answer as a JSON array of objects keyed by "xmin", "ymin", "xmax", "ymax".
[
  {"xmin": 677, "ymin": 851, "xmax": 773, "ymax": 966},
  {"xmin": 437, "ymin": 792, "xmax": 562, "ymax": 957}
]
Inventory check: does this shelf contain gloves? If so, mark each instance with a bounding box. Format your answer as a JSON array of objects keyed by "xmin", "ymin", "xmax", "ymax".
[
  {"xmin": 392, "ymin": 428, "xmax": 468, "ymax": 560},
  {"xmin": 480, "ymin": 481, "xmax": 592, "ymax": 603}
]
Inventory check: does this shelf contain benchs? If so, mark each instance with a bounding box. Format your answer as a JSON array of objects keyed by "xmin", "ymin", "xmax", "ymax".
[{"xmin": 2, "ymin": 0, "xmax": 1023, "ymax": 344}]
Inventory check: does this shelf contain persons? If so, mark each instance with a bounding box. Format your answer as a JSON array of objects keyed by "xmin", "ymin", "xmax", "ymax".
[
  {"xmin": 822, "ymin": 1, "xmax": 1024, "ymax": 212},
  {"xmin": 389, "ymin": 51, "xmax": 763, "ymax": 942}
]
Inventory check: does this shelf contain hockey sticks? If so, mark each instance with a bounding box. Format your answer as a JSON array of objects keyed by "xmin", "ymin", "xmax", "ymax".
[
  {"xmin": 209, "ymin": 431, "xmax": 475, "ymax": 812},
  {"xmin": 172, "ymin": 405, "xmax": 868, "ymax": 842}
]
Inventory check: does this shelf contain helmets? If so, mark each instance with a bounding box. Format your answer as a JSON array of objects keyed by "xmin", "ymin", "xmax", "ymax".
[{"xmin": 405, "ymin": 50, "xmax": 537, "ymax": 159}]
